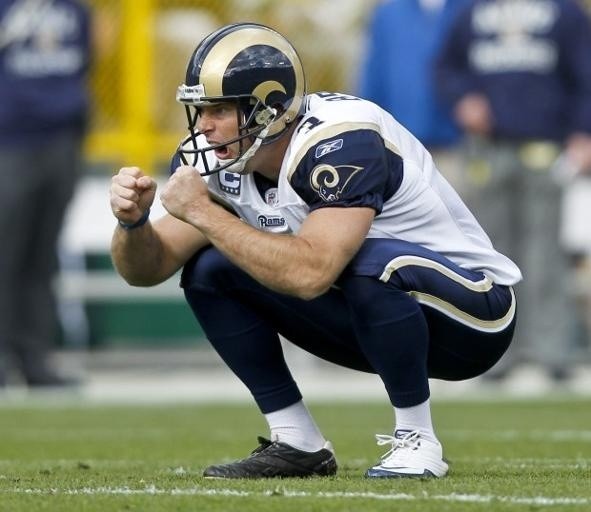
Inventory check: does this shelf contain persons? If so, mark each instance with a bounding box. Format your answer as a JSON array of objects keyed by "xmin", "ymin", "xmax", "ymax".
[
  {"xmin": 0, "ymin": 0, "xmax": 97, "ymax": 389},
  {"xmin": 355, "ymin": 0, "xmax": 469, "ymax": 188},
  {"xmin": 433, "ymin": 0, "xmax": 591, "ymax": 383},
  {"xmin": 107, "ymin": 22, "xmax": 524, "ymax": 484}
]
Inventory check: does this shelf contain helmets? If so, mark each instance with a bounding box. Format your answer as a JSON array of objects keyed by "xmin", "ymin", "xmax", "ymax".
[{"xmin": 175, "ymin": 21, "xmax": 308, "ymax": 146}]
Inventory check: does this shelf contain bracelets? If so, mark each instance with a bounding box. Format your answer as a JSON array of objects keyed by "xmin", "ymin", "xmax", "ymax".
[{"xmin": 117, "ymin": 206, "xmax": 151, "ymax": 231}]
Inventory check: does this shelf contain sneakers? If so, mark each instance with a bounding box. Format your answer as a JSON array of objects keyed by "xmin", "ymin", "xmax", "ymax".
[
  {"xmin": 203, "ymin": 436, "xmax": 338, "ymax": 478},
  {"xmin": 363, "ymin": 430, "xmax": 448, "ymax": 478}
]
